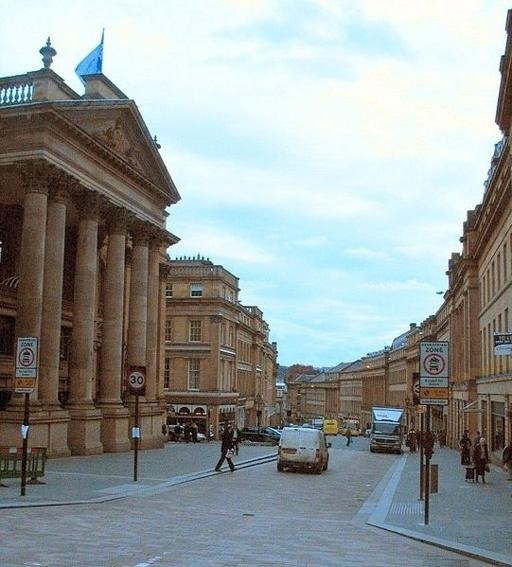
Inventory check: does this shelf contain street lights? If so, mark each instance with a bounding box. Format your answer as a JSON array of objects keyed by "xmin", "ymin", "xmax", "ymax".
[{"xmin": 256, "ymin": 393, "xmax": 262, "ymax": 425}]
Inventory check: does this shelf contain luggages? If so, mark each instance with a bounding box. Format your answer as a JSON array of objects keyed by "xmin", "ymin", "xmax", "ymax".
[{"xmin": 464, "ymin": 462, "xmax": 474, "ymax": 483}]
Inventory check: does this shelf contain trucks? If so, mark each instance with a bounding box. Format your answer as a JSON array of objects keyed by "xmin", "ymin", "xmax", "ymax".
[{"xmin": 369, "ymin": 405, "xmax": 407, "ymax": 455}]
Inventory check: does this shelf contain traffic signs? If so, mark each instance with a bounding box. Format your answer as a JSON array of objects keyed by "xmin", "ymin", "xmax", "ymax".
[
  {"xmin": 412, "ymin": 372, "xmax": 420, "ymax": 406},
  {"xmin": 127, "ymin": 364, "xmax": 146, "ymax": 397}
]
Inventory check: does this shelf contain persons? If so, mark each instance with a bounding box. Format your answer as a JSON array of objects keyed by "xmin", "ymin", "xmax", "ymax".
[
  {"xmin": 174, "ymin": 421, "xmax": 197, "ymax": 444},
  {"xmin": 502, "ymin": 440, "xmax": 512, "ymax": 481},
  {"xmin": 407, "ymin": 428, "xmax": 445, "ymax": 451},
  {"xmin": 460, "ymin": 433, "xmax": 471, "ymax": 465},
  {"xmin": 344, "ymin": 425, "xmax": 351, "ymax": 443},
  {"xmin": 215, "ymin": 425, "xmax": 235, "ymax": 472},
  {"xmin": 472, "ymin": 436, "xmax": 488, "ymax": 482},
  {"xmin": 230, "ymin": 424, "xmax": 244, "ymax": 455},
  {"xmin": 474, "ymin": 430, "xmax": 482, "ymax": 444}
]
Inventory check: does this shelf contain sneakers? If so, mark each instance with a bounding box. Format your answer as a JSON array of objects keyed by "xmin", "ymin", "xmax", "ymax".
[
  {"xmin": 476, "ymin": 479, "xmax": 487, "ymax": 485},
  {"xmin": 213, "ymin": 467, "xmax": 238, "ymax": 473}
]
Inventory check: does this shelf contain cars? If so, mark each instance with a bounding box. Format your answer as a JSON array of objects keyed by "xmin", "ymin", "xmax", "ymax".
[
  {"xmin": 167, "ymin": 424, "xmax": 207, "ymax": 443},
  {"xmin": 241, "ymin": 417, "xmax": 373, "ymax": 442}
]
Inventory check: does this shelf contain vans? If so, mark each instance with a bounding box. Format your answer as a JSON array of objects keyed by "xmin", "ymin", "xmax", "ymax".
[{"xmin": 276, "ymin": 424, "xmax": 332, "ymax": 475}]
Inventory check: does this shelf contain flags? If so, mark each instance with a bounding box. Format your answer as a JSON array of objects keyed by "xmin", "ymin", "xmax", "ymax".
[{"xmin": 75, "ymin": 30, "xmax": 105, "ymax": 84}]
